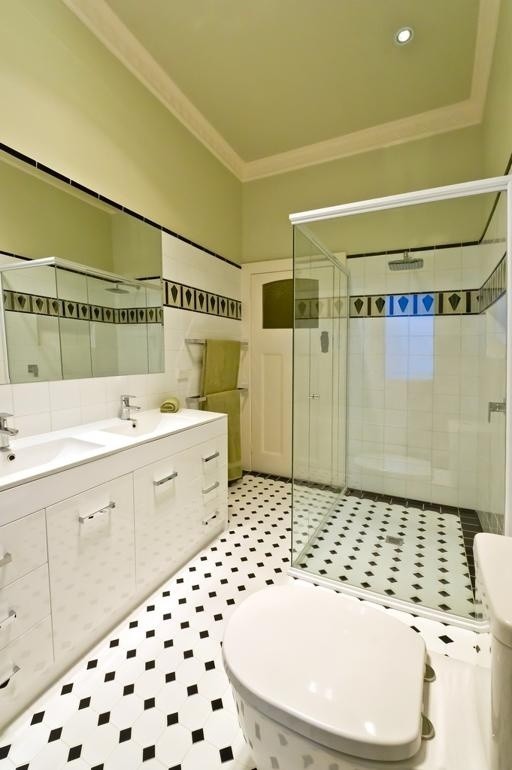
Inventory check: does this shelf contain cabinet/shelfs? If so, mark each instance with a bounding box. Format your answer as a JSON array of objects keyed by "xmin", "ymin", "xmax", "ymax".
[
  {"xmin": 134, "ymin": 426, "xmax": 190, "ymax": 609},
  {"xmin": 189, "ymin": 414, "xmax": 230, "ymax": 564},
  {"xmin": 0, "ymin": 476, "xmax": 54, "ymax": 736},
  {"xmin": 46, "ymin": 446, "xmax": 135, "ymax": 683}
]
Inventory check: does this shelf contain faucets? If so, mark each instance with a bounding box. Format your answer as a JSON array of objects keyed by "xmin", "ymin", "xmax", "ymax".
[
  {"xmin": 0, "ymin": 411, "xmax": 19, "ymax": 450},
  {"xmin": 119, "ymin": 394, "xmax": 141, "ymax": 420}
]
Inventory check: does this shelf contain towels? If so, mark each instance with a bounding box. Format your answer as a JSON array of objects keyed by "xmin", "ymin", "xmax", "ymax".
[
  {"xmin": 201, "ymin": 388, "xmax": 244, "ymax": 485},
  {"xmin": 157, "ymin": 395, "xmax": 181, "ymax": 415},
  {"xmin": 199, "ymin": 338, "xmax": 241, "ymax": 412}
]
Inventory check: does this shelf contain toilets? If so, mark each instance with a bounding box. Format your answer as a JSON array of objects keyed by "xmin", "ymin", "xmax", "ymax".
[{"xmin": 219, "ymin": 531, "xmax": 512, "ymax": 770}]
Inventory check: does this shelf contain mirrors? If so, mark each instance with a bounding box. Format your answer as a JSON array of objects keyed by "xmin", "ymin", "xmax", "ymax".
[{"xmin": 0, "ymin": 143, "xmax": 166, "ymax": 389}]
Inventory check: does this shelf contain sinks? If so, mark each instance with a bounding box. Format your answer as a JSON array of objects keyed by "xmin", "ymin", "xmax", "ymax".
[
  {"xmin": 0, "ymin": 436, "xmax": 106, "ymax": 480},
  {"xmin": 97, "ymin": 413, "xmax": 189, "ymax": 439}
]
